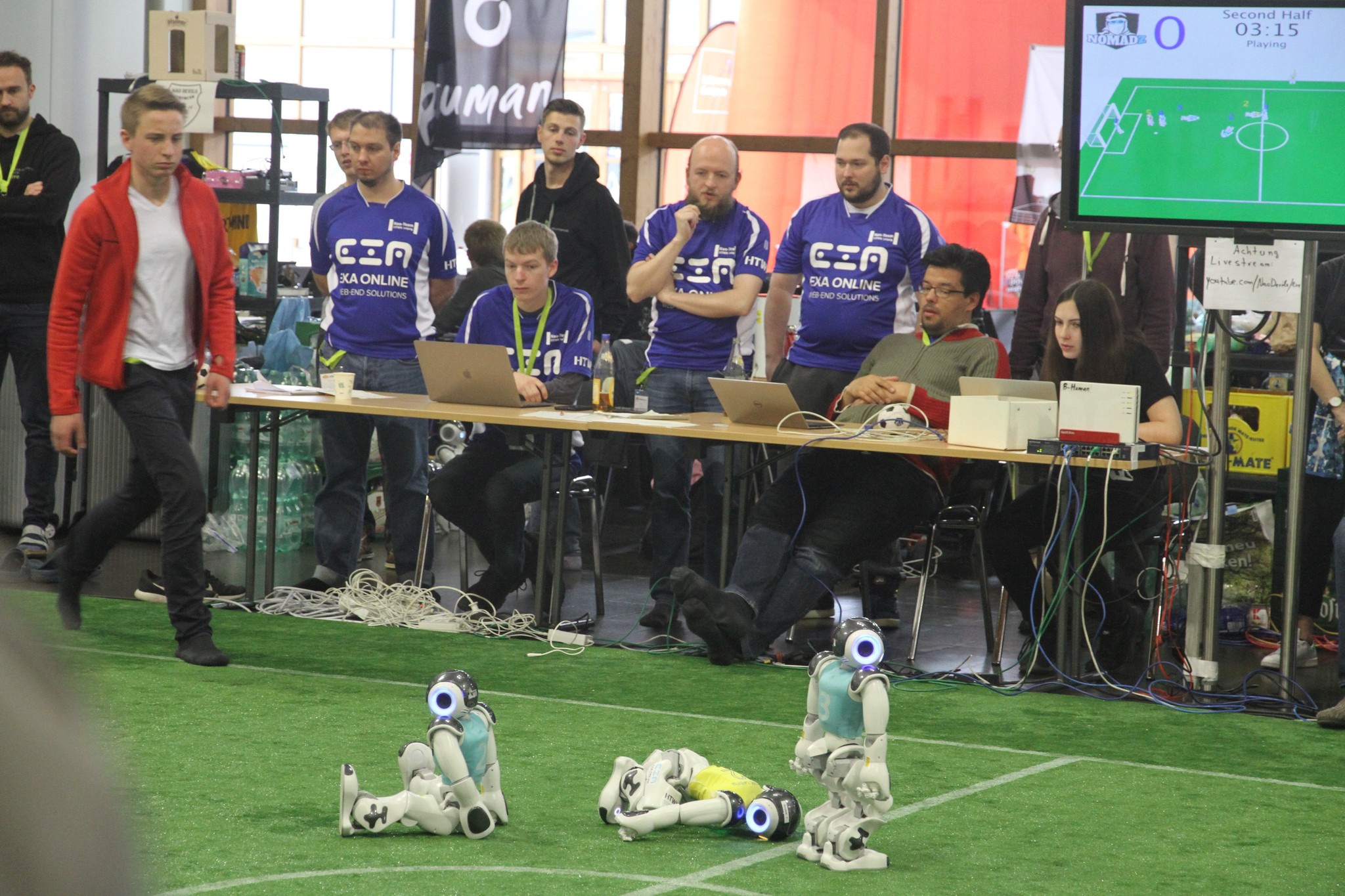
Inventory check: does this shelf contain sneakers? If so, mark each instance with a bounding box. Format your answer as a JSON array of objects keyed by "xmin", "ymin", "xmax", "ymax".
[
  {"xmin": 532, "ymin": 575, "xmax": 565, "ymax": 624},
  {"xmin": 1261, "ymin": 628, "xmax": 1318, "ymax": 670},
  {"xmin": 134, "ymin": 568, "xmax": 245, "ymax": 603},
  {"xmin": 457, "ymin": 565, "xmax": 527, "ymax": 613}
]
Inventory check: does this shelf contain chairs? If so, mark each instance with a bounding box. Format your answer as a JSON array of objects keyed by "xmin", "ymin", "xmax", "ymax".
[
  {"xmin": 414, "ymin": 426, "xmax": 609, "ymax": 620},
  {"xmin": 872, "ymin": 462, "xmax": 1005, "ymax": 663}
]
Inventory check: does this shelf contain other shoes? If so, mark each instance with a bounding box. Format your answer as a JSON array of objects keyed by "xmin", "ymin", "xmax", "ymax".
[
  {"xmin": 1316, "ymin": 697, "xmax": 1345, "ymax": 730},
  {"xmin": 18, "ymin": 524, "xmax": 48, "ymax": 558}
]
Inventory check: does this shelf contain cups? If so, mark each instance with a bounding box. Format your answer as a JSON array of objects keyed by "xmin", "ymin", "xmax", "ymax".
[{"xmin": 333, "ymin": 372, "xmax": 356, "ymax": 400}]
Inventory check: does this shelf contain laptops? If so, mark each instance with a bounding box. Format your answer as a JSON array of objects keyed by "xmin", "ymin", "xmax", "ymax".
[
  {"xmin": 958, "ymin": 375, "xmax": 1060, "ymax": 435},
  {"xmin": 413, "ymin": 340, "xmax": 557, "ymax": 408},
  {"xmin": 707, "ymin": 377, "xmax": 846, "ymax": 430}
]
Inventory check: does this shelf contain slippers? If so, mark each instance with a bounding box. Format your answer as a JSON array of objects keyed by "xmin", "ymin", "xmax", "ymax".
[{"xmin": 0, "ymin": 546, "xmax": 59, "ymax": 584}]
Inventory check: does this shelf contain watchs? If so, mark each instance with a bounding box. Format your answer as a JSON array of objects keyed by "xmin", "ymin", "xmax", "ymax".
[{"xmin": 1326, "ymin": 394, "xmax": 1344, "ymax": 412}]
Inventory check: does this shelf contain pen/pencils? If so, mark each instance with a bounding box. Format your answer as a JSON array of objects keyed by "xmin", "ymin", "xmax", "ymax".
[
  {"xmin": 560, "ymin": 411, "xmax": 563, "ymax": 415},
  {"xmin": 607, "ymin": 415, "xmax": 611, "ymax": 418}
]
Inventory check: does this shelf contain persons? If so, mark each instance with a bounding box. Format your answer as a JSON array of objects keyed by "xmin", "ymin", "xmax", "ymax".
[
  {"xmin": 599, "ymin": 746, "xmax": 803, "ymax": 841},
  {"xmin": 294, "ymin": 107, "xmax": 1345, "ymax": 727},
  {"xmin": 340, "ymin": 668, "xmax": 508, "ymax": 839},
  {"xmin": 46, "ymin": 84, "xmax": 235, "ymax": 665},
  {"xmin": 788, "ymin": 616, "xmax": 894, "ymax": 872},
  {"xmin": 1, "ymin": 50, "xmax": 81, "ymax": 559}
]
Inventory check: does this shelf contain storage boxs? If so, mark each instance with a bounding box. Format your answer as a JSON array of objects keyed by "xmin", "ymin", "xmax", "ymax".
[
  {"xmin": 144, "ymin": 11, "xmax": 237, "ymax": 82},
  {"xmin": 946, "ymin": 392, "xmax": 1060, "ymax": 453}
]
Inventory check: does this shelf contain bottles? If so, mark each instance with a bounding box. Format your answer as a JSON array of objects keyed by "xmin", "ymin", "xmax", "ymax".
[
  {"xmin": 275, "ymin": 452, "xmax": 302, "ymax": 552},
  {"xmin": 592, "ymin": 334, "xmax": 615, "ymax": 412},
  {"xmin": 302, "ymin": 456, "xmax": 323, "ymax": 548},
  {"xmin": 270, "ymin": 370, "xmax": 323, "ymax": 462},
  {"xmin": 228, "ymin": 456, "xmax": 269, "ymax": 551},
  {"xmin": 231, "ymin": 368, "xmax": 271, "ymax": 458}
]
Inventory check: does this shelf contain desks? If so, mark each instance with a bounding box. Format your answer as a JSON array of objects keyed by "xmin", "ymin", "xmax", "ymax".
[{"xmin": 194, "ymin": 381, "xmax": 1209, "ymax": 688}]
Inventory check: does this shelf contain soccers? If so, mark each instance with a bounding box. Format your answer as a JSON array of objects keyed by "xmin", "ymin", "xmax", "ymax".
[{"xmin": 877, "ymin": 404, "xmax": 911, "ymax": 436}]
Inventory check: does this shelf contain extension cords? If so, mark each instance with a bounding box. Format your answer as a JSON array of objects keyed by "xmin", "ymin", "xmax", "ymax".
[
  {"xmin": 339, "ymin": 594, "xmax": 371, "ymax": 620},
  {"xmin": 418, "ymin": 619, "xmax": 464, "ymax": 633},
  {"xmin": 548, "ymin": 629, "xmax": 594, "ymax": 647}
]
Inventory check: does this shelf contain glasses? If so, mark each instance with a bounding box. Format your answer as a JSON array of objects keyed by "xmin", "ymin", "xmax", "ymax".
[
  {"xmin": 918, "ymin": 283, "xmax": 964, "ymax": 298},
  {"xmin": 329, "ymin": 138, "xmax": 350, "ymax": 151}
]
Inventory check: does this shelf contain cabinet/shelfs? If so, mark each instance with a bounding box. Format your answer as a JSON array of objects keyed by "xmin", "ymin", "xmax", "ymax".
[
  {"xmin": 1163, "ymin": 238, "xmax": 1296, "ymax": 646},
  {"xmin": 95, "ymin": 75, "xmax": 330, "ymax": 367}
]
